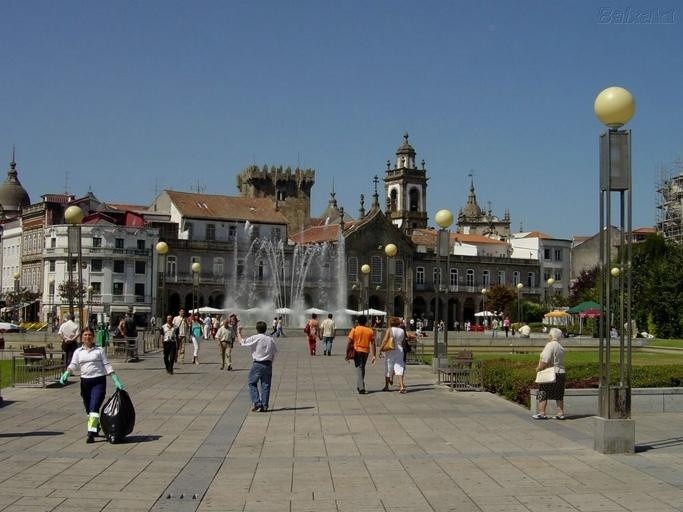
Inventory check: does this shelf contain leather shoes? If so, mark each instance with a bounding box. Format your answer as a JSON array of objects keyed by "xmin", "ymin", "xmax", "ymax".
[{"xmin": 252, "ymin": 405, "xmax": 262, "ymax": 411}]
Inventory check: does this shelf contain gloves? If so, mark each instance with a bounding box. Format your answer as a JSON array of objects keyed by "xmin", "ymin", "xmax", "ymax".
[
  {"xmin": 112, "ymin": 374, "xmax": 123, "ymax": 390},
  {"xmin": 60, "ymin": 370, "xmax": 70, "ymax": 385}
]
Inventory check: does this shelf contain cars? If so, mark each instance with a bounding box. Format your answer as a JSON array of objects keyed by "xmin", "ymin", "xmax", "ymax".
[{"xmin": 0, "ymin": 319, "xmax": 20, "ymax": 333}]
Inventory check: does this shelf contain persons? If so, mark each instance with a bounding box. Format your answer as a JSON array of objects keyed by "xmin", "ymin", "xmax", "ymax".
[
  {"xmin": 531, "ymin": 326, "xmax": 573, "ymax": 422},
  {"xmin": 57, "ymin": 326, "xmax": 123, "ymax": 446},
  {"xmin": 236, "ymin": 317, "xmax": 278, "ymax": 413},
  {"xmin": 150, "ymin": 308, "xmax": 233, "ymax": 375},
  {"xmin": 59, "ymin": 312, "xmax": 139, "ymax": 377},
  {"xmin": 518, "ymin": 322, "xmax": 530, "ymax": 338},
  {"xmin": 305, "ymin": 313, "xmax": 336, "ymax": 356},
  {"xmin": 346, "ymin": 315, "xmax": 426, "ymax": 395},
  {"xmin": 432, "ymin": 316, "xmax": 510, "ymax": 338},
  {"xmin": 270, "ymin": 316, "xmax": 288, "ymax": 338}
]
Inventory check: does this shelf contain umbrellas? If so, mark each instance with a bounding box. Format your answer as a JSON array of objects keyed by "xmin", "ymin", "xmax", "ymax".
[{"xmin": 565, "ymin": 299, "xmax": 612, "ymax": 314}]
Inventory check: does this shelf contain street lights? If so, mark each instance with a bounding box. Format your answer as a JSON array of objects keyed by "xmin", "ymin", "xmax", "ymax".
[
  {"xmin": 384, "ymin": 242, "xmax": 398, "ymax": 330},
  {"xmin": 592, "ymin": 85, "xmax": 637, "ymax": 421},
  {"xmin": 435, "ymin": 210, "xmax": 453, "ymax": 359},
  {"xmin": 481, "ymin": 267, "xmax": 620, "ymax": 331},
  {"xmin": 192, "ymin": 263, "xmax": 200, "ymax": 323},
  {"xmin": 156, "ymin": 241, "xmax": 169, "ymax": 326},
  {"xmin": 64, "ymin": 204, "xmax": 85, "ymax": 339},
  {"xmin": 361, "ymin": 263, "xmax": 371, "ymax": 326}
]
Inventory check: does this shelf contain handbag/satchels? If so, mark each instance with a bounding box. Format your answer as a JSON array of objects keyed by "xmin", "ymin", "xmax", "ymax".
[
  {"xmin": 347, "ymin": 326, "xmax": 356, "ymax": 358},
  {"xmin": 534, "ymin": 366, "xmax": 556, "ymax": 384},
  {"xmin": 382, "ymin": 327, "xmax": 395, "ymax": 352},
  {"xmin": 304, "ymin": 320, "xmax": 310, "ymax": 333}
]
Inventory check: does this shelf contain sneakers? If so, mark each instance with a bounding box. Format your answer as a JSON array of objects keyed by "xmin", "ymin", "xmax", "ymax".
[
  {"xmin": 552, "ymin": 415, "xmax": 564, "ymax": 420},
  {"xmin": 87, "ymin": 437, "xmax": 94, "ymax": 443},
  {"xmin": 531, "ymin": 414, "xmax": 547, "ymax": 420}
]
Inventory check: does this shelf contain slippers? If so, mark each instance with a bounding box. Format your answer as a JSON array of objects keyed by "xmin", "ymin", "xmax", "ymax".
[{"xmin": 399, "ymin": 388, "xmax": 406, "ymax": 393}]
